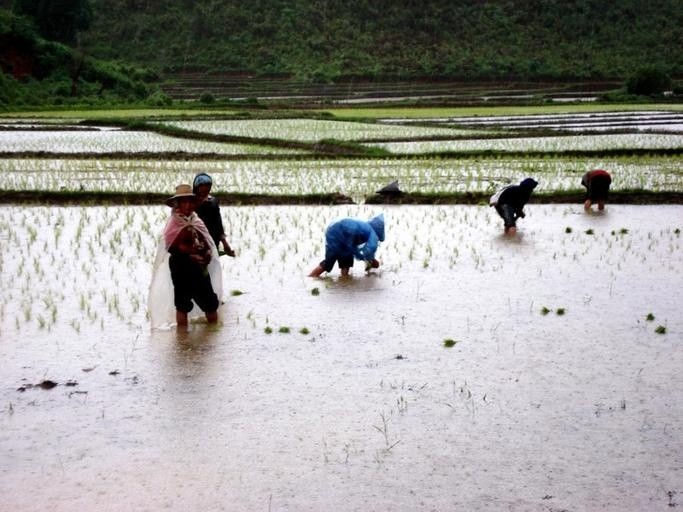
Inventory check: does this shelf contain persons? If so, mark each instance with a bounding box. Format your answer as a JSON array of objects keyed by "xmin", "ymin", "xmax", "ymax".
[
  {"xmin": 494, "ymin": 177, "xmax": 539, "ymax": 237},
  {"xmin": 160, "ymin": 183, "xmax": 219, "ymax": 327},
  {"xmin": 304, "ymin": 212, "xmax": 385, "ymax": 281},
  {"xmin": 580, "ymin": 169, "xmax": 611, "ymax": 211},
  {"xmin": 190, "ymin": 171, "xmax": 235, "ymax": 258}
]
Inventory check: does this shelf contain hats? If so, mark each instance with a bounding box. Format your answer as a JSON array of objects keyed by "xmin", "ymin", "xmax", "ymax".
[{"xmin": 165, "ymin": 185, "xmax": 200, "ymax": 207}]
